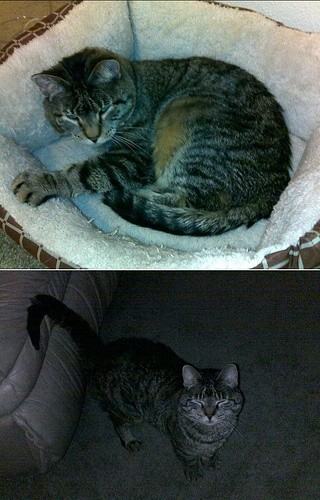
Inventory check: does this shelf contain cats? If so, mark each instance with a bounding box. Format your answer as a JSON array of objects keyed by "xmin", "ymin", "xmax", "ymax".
[
  {"xmin": 12, "ymin": 45, "xmax": 293, "ymax": 236},
  {"xmin": 27, "ymin": 294, "xmax": 244, "ymax": 484}
]
